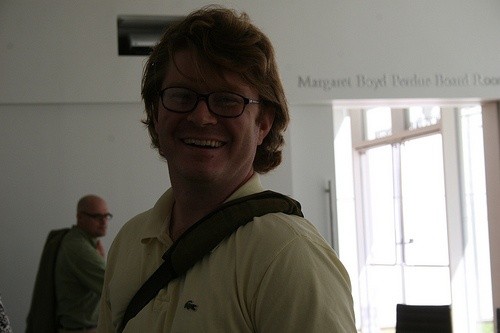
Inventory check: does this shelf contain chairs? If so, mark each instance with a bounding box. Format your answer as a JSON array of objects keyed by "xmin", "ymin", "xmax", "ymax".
[{"xmin": 396, "ymin": 302, "xmax": 452, "ymax": 333}]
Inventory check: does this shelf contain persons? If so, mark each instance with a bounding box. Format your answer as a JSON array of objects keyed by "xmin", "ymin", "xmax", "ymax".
[
  {"xmin": 53, "ymin": 195, "xmax": 111, "ymax": 333},
  {"xmin": 88, "ymin": 7, "xmax": 357, "ymax": 333}
]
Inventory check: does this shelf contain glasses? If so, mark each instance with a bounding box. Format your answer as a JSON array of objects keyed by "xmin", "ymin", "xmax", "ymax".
[
  {"xmin": 158, "ymin": 86, "xmax": 270, "ymax": 117},
  {"xmin": 79, "ymin": 211, "xmax": 113, "ymax": 221}
]
